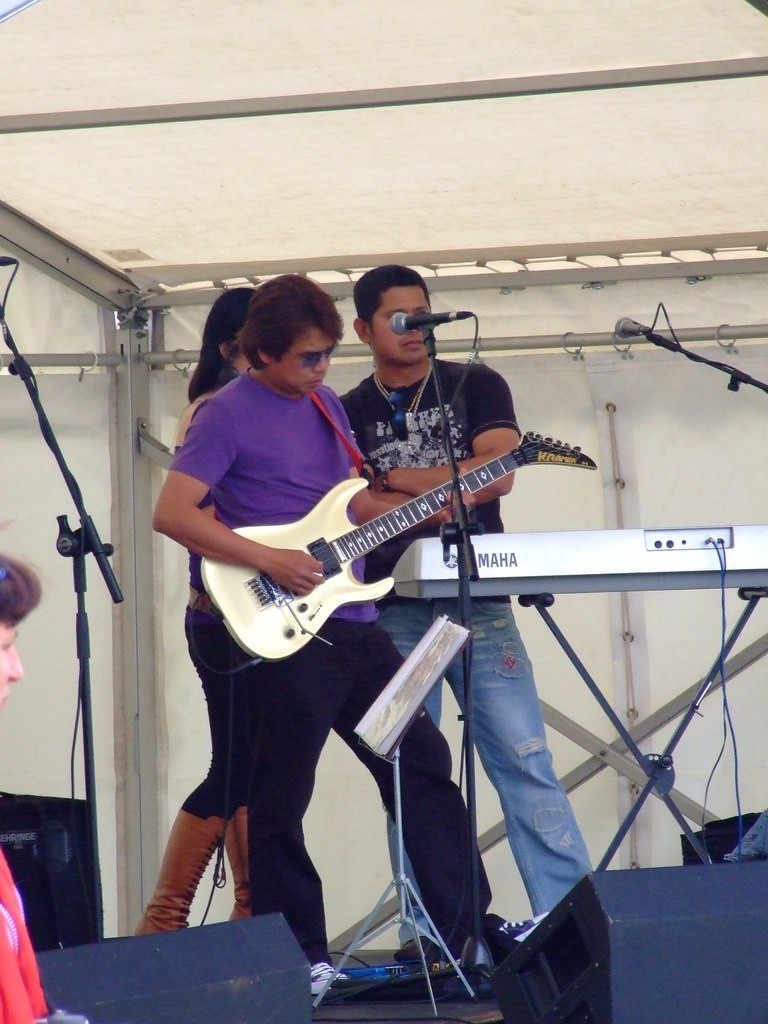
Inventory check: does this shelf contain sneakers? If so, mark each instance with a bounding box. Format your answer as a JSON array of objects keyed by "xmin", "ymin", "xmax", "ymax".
[
  {"xmin": 499, "ymin": 912, "xmax": 550, "ymax": 942},
  {"xmin": 310, "ymin": 962, "xmax": 351, "ymax": 994}
]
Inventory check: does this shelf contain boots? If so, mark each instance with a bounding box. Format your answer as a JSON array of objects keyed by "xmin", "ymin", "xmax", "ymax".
[
  {"xmin": 135, "ymin": 807, "xmax": 226, "ymax": 937},
  {"xmin": 224, "ymin": 806, "xmax": 251, "ymax": 922}
]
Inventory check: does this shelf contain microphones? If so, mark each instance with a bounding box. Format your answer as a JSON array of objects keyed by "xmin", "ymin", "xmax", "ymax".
[
  {"xmin": 389, "ymin": 312, "xmax": 474, "ymax": 335},
  {"xmin": 0, "ymin": 256, "xmax": 17, "ymax": 266},
  {"xmin": 614, "ymin": 317, "xmax": 650, "ymax": 338}
]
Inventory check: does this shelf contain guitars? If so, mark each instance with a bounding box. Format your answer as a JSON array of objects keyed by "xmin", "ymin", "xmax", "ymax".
[{"xmin": 199, "ymin": 434, "xmax": 600, "ymax": 660}]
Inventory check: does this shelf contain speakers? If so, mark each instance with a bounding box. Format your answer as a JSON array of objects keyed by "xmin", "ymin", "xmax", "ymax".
[
  {"xmin": 0, "ymin": 793, "xmax": 313, "ymax": 1024},
  {"xmin": 489, "ymin": 858, "xmax": 768, "ymax": 1024}
]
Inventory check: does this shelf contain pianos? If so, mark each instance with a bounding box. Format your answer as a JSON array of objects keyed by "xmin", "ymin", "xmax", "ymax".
[{"xmin": 389, "ymin": 521, "xmax": 767, "ymax": 601}]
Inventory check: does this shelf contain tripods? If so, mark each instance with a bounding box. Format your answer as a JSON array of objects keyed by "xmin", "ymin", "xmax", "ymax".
[{"xmin": 312, "ymin": 615, "xmax": 479, "ymax": 1016}]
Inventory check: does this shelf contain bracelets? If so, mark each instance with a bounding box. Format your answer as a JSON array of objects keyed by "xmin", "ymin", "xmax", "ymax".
[{"xmin": 382, "ymin": 465, "xmax": 398, "ymax": 491}]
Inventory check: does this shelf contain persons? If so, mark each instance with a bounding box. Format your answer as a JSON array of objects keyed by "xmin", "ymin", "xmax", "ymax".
[
  {"xmin": 137, "ymin": 288, "xmax": 265, "ymax": 935},
  {"xmin": 338, "ymin": 264, "xmax": 593, "ymax": 966},
  {"xmin": 0, "ymin": 552, "xmax": 50, "ymax": 1024},
  {"xmin": 153, "ymin": 273, "xmax": 552, "ymax": 994}
]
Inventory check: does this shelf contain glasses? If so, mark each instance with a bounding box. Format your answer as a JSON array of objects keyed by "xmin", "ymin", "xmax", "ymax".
[{"xmin": 282, "ymin": 343, "xmax": 336, "ymax": 367}]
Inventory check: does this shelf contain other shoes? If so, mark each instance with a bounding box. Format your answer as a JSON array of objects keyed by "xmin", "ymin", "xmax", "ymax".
[{"xmin": 394, "ymin": 937, "xmax": 438, "ymax": 962}]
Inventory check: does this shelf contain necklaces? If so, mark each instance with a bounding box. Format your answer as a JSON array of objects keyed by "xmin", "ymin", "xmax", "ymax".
[{"xmin": 374, "ymin": 357, "xmax": 433, "ymax": 434}]
